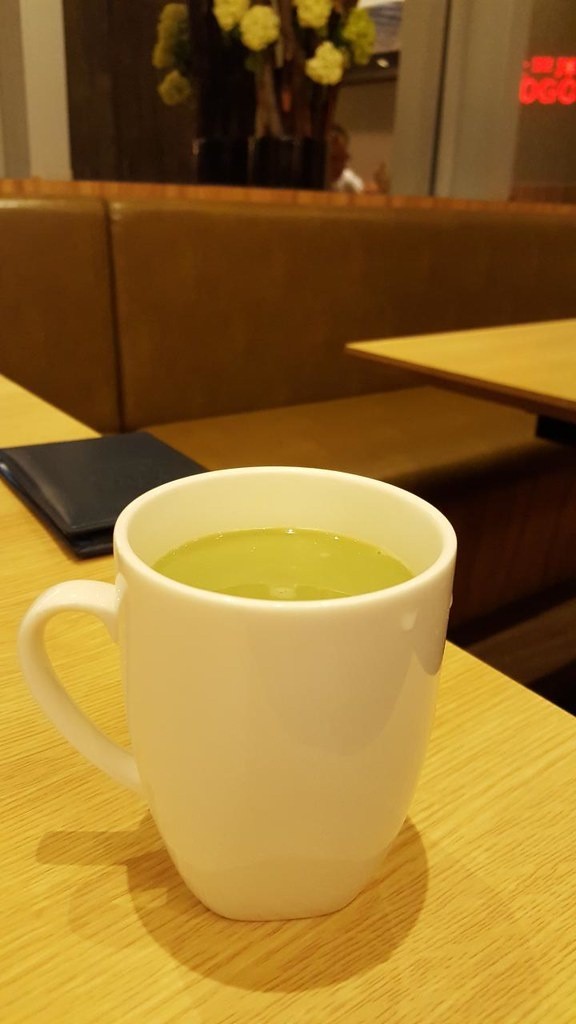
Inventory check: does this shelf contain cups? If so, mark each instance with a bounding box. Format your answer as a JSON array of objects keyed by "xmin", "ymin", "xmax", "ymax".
[{"xmin": 19, "ymin": 465, "xmax": 459, "ymax": 921}]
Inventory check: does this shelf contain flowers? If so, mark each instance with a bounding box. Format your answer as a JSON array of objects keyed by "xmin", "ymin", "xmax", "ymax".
[{"xmin": 152, "ymin": 0, "xmax": 375, "ymax": 188}]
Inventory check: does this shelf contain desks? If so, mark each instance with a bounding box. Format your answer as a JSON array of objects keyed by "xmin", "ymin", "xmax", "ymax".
[
  {"xmin": 346, "ymin": 317, "xmax": 576, "ymax": 446},
  {"xmin": 0, "ymin": 375, "xmax": 576, "ymax": 1024}
]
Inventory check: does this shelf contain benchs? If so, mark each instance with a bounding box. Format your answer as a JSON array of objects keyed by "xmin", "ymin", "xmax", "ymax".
[{"xmin": 0, "ymin": 177, "xmax": 576, "ymax": 634}]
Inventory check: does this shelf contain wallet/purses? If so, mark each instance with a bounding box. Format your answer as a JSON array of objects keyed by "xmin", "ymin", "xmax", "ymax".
[{"xmin": 0, "ymin": 432, "xmax": 214, "ymax": 559}]
